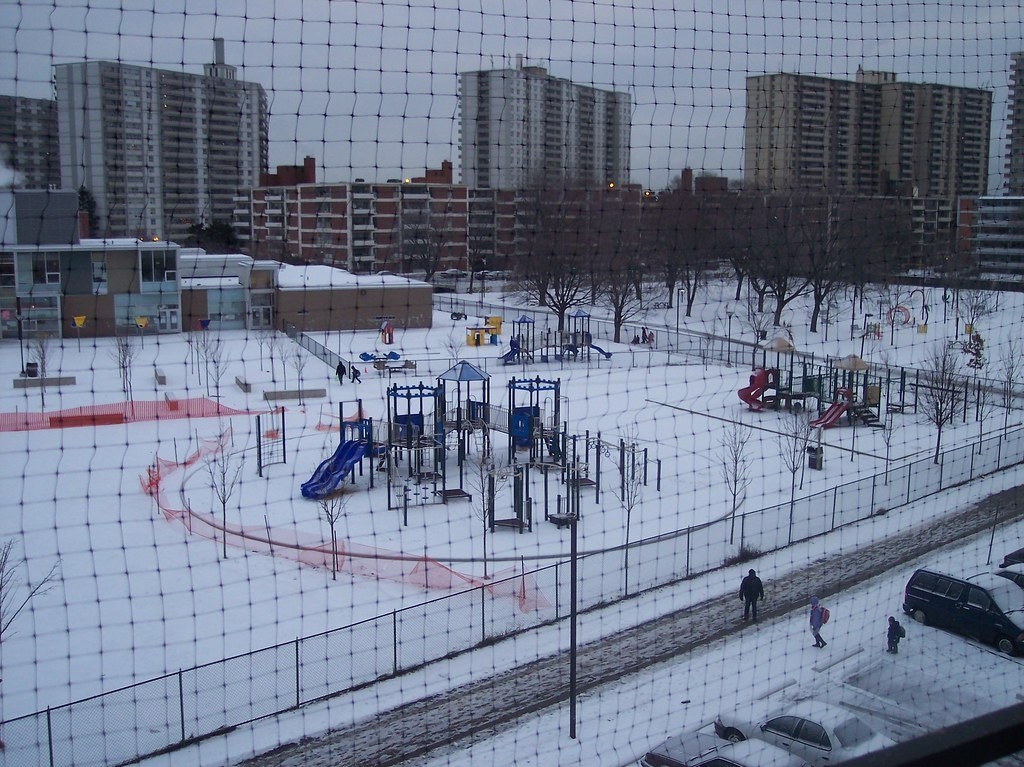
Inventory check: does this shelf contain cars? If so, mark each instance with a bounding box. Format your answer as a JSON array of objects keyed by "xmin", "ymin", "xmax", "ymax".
[
  {"xmin": 990, "ymin": 548, "xmax": 1024, "ymax": 589},
  {"xmin": 642, "ymin": 699, "xmax": 899, "ymax": 767}
]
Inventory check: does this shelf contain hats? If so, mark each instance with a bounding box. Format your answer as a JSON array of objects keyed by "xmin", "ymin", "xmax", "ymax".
[{"xmin": 811, "ymin": 597, "xmax": 818, "ymax": 604}]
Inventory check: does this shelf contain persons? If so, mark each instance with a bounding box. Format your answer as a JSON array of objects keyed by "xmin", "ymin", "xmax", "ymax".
[
  {"xmin": 350, "ymin": 365, "xmax": 362, "ymax": 384},
  {"xmin": 885, "ymin": 615, "xmax": 900, "ymax": 654},
  {"xmin": 809, "ymin": 596, "xmax": 827, "ymax": 649},
  {"xmin": 739, "ymin": 568, "xmax": 764, "ymax": 622},
  {"xmin": 631, "ymin": 326, "xmax": 655, "ymax": 344},
  {"xmin": 335, "ymin": 361, "xmax": 346, "ymax": 385}
]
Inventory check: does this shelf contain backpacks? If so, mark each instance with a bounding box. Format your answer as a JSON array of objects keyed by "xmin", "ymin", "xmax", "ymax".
[
  {"xmin": 898, "ymin": 625, "xmax": 906, "ymax": 638},
  {"xmin": 813, "ymin": 606, "xmax": 830, "ymax": 624}
]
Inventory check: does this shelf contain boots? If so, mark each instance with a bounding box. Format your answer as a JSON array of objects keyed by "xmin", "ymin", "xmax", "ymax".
[
  {"xmin": 887, "ymin": 647, "xmax": 898, "ymax": 654},
  {"xmin": 812, "ymin": 634, "xmax": 827, "ymax": 648}
]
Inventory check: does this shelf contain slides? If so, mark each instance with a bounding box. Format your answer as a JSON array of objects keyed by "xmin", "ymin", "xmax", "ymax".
[
  {"xmin": 738, "ymin": 383, "xmax": 765, "ymax": 411},
  {"xmin": 590, "ymin": 345, "xmax": 612, "ymax": 358},
  {"xmin": 497, "ymin": 348, "xmax": 519, "ymax": 365},
  {"xmin": 808, "ymin": 401, "xmax": 848, "ymax": 428},
  {"xmin": 364, "ymin": 443, "xmax": 386, "ymax": 457},
  {"xmin": 299, "ymin": 439, "xmax": 367, "ymax": 499}
]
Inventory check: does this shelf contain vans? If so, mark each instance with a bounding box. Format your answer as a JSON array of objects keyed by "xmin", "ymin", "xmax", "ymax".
[{"xmin": 902, "ymin": 563, "xmax": 1024, "ymax": 656}]
